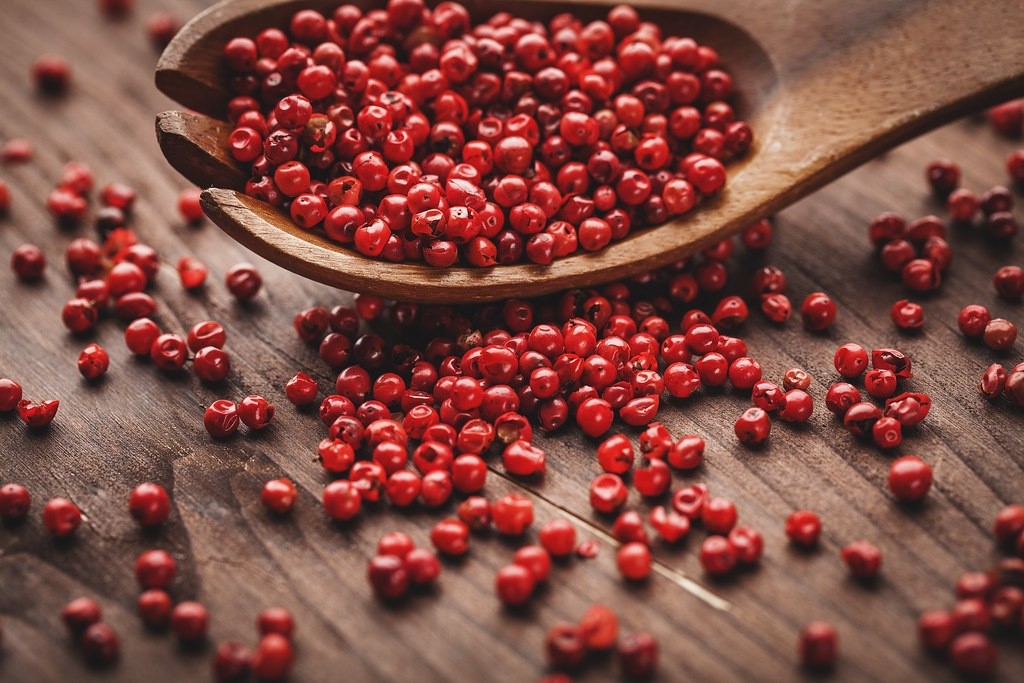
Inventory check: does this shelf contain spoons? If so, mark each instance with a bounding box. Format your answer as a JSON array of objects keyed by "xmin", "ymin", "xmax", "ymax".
[{"xmin": 152, "ymin": 0, "xmax": 1024, "ymax": 301}]
